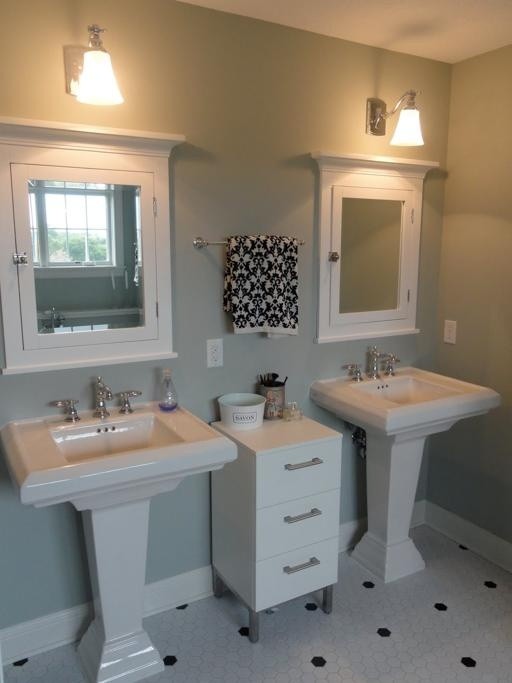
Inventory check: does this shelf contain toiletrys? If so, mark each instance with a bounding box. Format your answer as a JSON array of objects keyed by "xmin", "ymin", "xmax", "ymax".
[{"xmin": 282, "ymin": 401, "xmax": 304, "ymax": 422}]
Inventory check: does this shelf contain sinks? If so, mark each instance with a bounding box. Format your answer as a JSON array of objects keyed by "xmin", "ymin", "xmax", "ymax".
[
  {"xmin": 0, "ymin": 401, "xmax": 236, "ymax": 509},
  {"xmin": 309, "ymin": 366, "xmax": 500, "ymax": 434}
]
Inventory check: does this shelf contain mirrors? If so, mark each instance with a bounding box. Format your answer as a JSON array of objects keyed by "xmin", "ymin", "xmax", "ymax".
[
  {"xmin": 0, "ymin": 114, "xmax": 188, "ymax": 376},
  {"xmin": 307, "ymin": 148, "xmax": 442, "ymax": 348}
]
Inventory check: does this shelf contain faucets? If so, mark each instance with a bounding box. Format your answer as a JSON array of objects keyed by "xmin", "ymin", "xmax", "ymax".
[
  {"xmin": 366, "ymin": 344, "xmax": 389, "ymax": 381},
  {"xmin": 93, "ymin": 375, "xmax": 111, "ymax": 417},
  {"xmin": 48, "ymin": 306, "xmax": 72, "ymax": 329}
]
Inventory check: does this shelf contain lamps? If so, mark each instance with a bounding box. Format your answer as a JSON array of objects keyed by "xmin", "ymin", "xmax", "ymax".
[
  {"xmin": 66, "ymin": 24, "xmax": 126, "ymax": 108},
  {"xmin": 365, "ymin": 88, "xmax": 426, "ymax": 147}
]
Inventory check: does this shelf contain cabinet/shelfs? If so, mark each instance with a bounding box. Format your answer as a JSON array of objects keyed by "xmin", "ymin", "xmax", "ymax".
[{"xmin": 209, "ymin": 414, "xmax": 348, "ymax": 646}]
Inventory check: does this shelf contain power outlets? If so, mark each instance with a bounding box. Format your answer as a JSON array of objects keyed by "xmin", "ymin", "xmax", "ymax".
[
  {"xmin": 441, "ymin": 318, "xmax": 459, "ymax": 345},
  {"xmin": 205, "ymin": 337, "xmax": 226, "ymax": 370}
]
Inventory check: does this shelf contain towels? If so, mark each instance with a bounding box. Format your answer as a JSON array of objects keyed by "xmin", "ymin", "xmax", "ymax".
[{"xmin": 219, "ymin": 233, "xmax": 302, "ymax": 337}]
